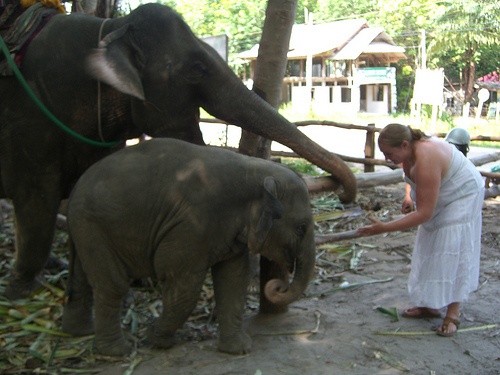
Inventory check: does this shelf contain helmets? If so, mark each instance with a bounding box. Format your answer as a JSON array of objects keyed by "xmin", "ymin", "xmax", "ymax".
[{"xmin": 444, "ymin": 128, "xmax": 471, "ymax": 148}]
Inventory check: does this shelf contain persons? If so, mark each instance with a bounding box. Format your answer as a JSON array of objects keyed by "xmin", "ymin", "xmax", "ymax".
[
  {"xmin": 358, "ymin": 123, "xmax": 484, "ymax": 337},
  {"xmin": 444, "ymin": 128, "xmax": 471, "ymax": 158}
]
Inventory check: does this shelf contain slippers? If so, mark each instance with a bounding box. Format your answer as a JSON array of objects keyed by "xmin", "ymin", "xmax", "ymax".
[
  {"xmin": 436, "ymin": 317, "xmax": 461, "ymax": 337},
  {"xmin": 402, "ymin": 306, "xmax": 442, "ymax": 319}
]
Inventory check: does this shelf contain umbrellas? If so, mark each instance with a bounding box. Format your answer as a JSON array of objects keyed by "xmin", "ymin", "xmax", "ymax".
[{"xmin": 477, "ymin": 71, "xmax": 500, "ymax": 87}]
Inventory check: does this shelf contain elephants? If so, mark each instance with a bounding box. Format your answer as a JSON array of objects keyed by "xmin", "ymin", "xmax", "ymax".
[{"xmin": 0, "ymin": 1, "xmax": 359, "ymax": 361}]
